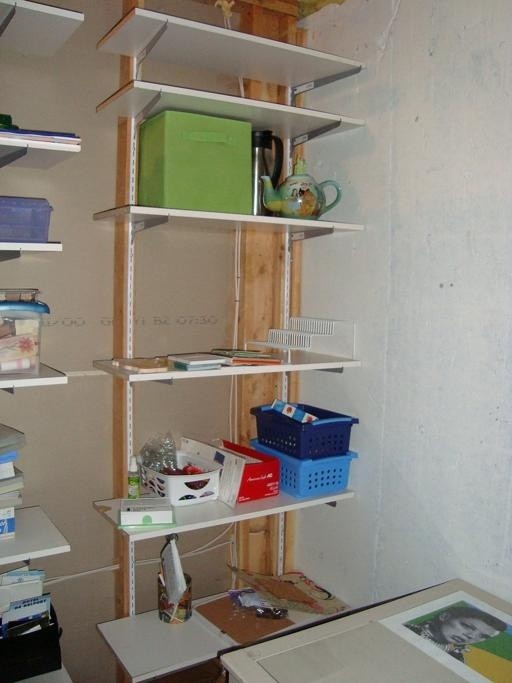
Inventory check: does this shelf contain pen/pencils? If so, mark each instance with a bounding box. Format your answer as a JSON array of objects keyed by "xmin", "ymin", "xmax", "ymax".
[{"xmin": 157, "ymin": 572, "xmax": 183, "ymax": 623}]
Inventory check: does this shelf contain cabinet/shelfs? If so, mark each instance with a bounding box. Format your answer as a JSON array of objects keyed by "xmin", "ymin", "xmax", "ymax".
[
  {"xmin": 93, "ymin": 0, "xmax": 366, "ymax": 683},
  {"xmin": 0, "ymin": 0, "xmax": 85, "ymax": 683}
]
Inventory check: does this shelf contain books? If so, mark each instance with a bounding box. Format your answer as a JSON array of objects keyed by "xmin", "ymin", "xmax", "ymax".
[
  {"xmin": 0, "ymin": 123, "xmax": 80, "ymax": 146},
  {"xmin": 111, "ymin": 347, "xmax": 282, "ymax": 373},
  {"xmin": 0, "ymin": 419, "xmax": 51, "ymax": 636}
]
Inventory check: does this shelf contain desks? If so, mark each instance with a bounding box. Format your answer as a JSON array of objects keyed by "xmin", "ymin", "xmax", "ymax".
[{"xmin": 221, "ymin": 579, "xmax": 512, "ymax": 683}]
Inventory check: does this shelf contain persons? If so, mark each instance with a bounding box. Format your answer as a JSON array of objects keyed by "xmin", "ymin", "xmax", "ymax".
[{"xmin": 404, "ymin": 605, "xmax": 508, "ymax": 666}]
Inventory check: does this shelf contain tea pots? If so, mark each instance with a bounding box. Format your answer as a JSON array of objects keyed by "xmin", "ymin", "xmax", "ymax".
[
  {"xmin": 251, "ymin": 127, "xmax": 284, "ymax": 214},
  {"xmin": 260, "ymin": 171, "xmax": 342, "ymax": 220}
]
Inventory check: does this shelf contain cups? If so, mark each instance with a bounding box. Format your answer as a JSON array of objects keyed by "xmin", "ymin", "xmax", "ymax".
[{"xmin": 155, "ymin": 572, "xmax": 192, "ymax": 622}]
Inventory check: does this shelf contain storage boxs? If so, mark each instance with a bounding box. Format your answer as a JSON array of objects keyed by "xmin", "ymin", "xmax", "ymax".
[
  {"xmin": 0, "ymin": 301, "xmax": 50, "ymax": 374},
  {"xmin": 0, "ymin": 196, "xmax": 53, "ymax": 243},
  {"xmin": 140, "ymin": 110, "xmax": 253, "ymax": 214},
  {"xmin": 210, "ymin": 439, "xmax": 279, "ymax": 503}
]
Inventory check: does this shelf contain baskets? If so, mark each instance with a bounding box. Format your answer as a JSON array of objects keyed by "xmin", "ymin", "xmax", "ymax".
[
  {"xmin": 249, "ymin": 437, "xmax": 359, "ymax": 500},
  {"xmin": 249, "ymin": 399, "xmax": 361, "ymax": 460},
  {"xmin": 137, "ymin": 451, "xmax": 224, "ymax": 508}
]
